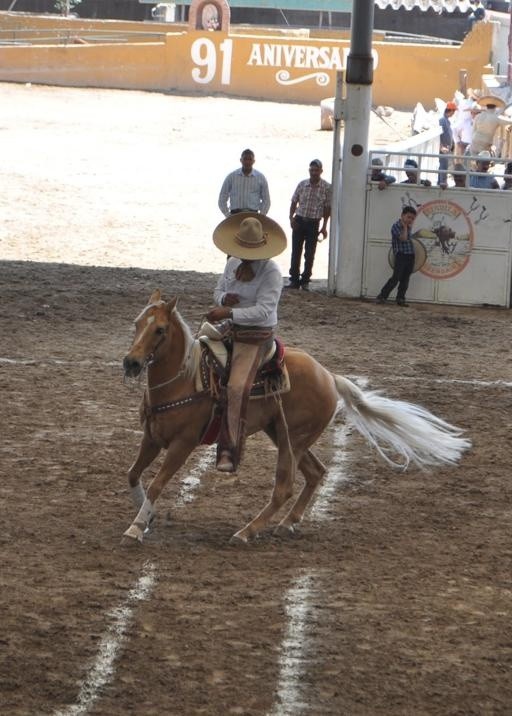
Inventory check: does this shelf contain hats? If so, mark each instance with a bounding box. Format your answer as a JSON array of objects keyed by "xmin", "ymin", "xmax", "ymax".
[
  {"xmin": 212, "ymin": 212, "xmax": 287, "ymax": 260},
  {"xmin": 310, "ymin": 159, "xmax": 321, "ymax": 167},
  {"xmin": 477, "ymin": 151, "xmax": 489, "ymax": 157},
  {"xmin": 478, "ymin": 95, "xmax": 506, "ymax": 108},
  {"xmin": 447, "ymin": 102, "xmax": 458, "ymax": 110}
]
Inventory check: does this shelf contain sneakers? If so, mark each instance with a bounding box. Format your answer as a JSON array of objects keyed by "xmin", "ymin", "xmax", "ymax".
[
  {"xmin": 376, "ymin": 297, "xmax": 409, "ymax": 306},
  {"xmin": 284, "ymin": 282, "xmax": 309, "ymax": 291},
  {"xmin": 217, "ymin": 451, "xmax": 233, "ymax": 471}
]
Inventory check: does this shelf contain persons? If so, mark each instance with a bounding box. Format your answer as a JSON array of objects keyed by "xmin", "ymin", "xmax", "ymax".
[
  {"xmin": 216, "ymin": 149, "xmax": 272, "ymax": 262},
  {"xmin": 280, "ymin": 158, "xmax": 333, "ymax": 291},
  {"xmin": 375, "ymin": 205, "xmax": 418, "ymax": 308},
  {"xmin": 202, "ymin": 210, "xmax": 288, "ymax": 475},
  {"xmin": 371, "ymin": 94, "xmax": 511, "ymax": 192}
]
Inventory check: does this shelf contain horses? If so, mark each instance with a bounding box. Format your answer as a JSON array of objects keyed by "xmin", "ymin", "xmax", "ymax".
[{"xmin": 118, "ymin": 286, "xmax": 473, "ymax": 549}]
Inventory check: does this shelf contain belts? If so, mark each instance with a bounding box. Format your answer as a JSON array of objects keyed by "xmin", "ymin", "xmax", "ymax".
[{"xmin": 296, "ymin": 215, "xmax": 320, "ymax": 223}]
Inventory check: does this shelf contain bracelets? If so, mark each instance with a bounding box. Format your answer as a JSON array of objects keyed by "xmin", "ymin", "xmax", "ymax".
[{"xmin": 228, "ymin": 310, "xmax": 233, "ymax": 318}]
